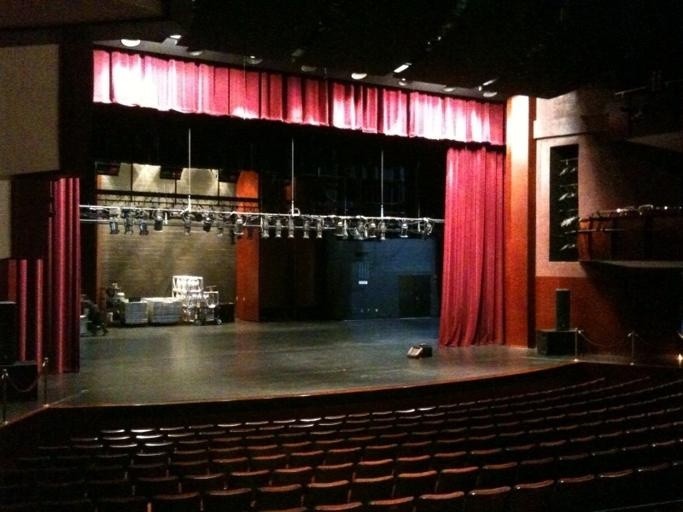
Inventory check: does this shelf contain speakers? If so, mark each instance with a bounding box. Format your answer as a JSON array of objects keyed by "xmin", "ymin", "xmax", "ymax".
[
  {"xmin": 536, "ymin": 328, "xmax": 581, "ymax": 356},
  {"xmin": 555, "ymin": 289, "xmax": 570, "ymax": 331},
  {"xmin": 2, "ymin": 361, "xmax": 38, "ymax": 403},
  {"xmin": 0, "ymin": 42, "xmax": 95, "ymax": 179},
  {"xmin": 0, "ymin": 301, "xmax": 18, "ymax": 363}
]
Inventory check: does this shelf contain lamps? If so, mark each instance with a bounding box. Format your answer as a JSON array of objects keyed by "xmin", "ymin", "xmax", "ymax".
[
  {"xmin": 75, "ymin": 204, "xmax": 444, "ymax": 241},
  {"xmin": 555, "ymin": 157, "xmax": 578, "ymax": 251}
]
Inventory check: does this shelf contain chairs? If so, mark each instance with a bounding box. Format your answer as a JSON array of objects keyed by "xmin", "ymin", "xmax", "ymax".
[{"xmin": 2, "ymin": 375, "xmax": 683, "ymax": 512}]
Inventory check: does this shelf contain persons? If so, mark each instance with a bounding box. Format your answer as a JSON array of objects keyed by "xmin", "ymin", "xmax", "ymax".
[{"xmin": 79, "ymin": 294, "xmax": 109, "ymax": 337}]
